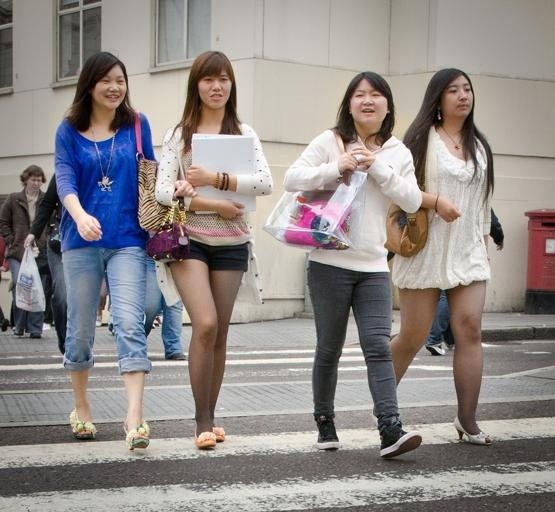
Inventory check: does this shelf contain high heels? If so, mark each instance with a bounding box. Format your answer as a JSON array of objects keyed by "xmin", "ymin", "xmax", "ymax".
[{"xmin": 453, "ymin": 414, "xmax": 491, "ymax": 447}]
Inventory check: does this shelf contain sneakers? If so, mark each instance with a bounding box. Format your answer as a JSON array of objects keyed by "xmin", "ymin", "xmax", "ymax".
[
  {"xmin": 213, "ymin": 427, "xmax": 227, "ymax": 442},
  {"xmin": 124, "ymin": 415, "xmax": 149, "ymax": 450},
  {"xmin": 2, "ymin": 319, "xmax": 44, "ymax": 340},
  {"xmin": 378, "ymin": 429, "xmax": 424, "ymax": 458},
  {"xmin": 69, "ymin": 408, "xmax": 95, "ymax": 440},
  {"xmin": 161, "ymin": 349, "xmax": 187, "ymax": 362},
  {"xmin": 424, "ymin": 342, "xmax": 447, "ymax": 358},
  {"xmin": 193, "ymin": 426, "xmax": 215, "ymax": 449},
  {"xmin": 314, "ymin": 414, "xmax": 340, "ymax": 450}
]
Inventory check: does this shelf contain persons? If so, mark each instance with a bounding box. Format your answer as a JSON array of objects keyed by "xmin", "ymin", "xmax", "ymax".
[
  {"xmin": 390, "ymin": 68, "xmax": 494, "ymax": 447},
  {"xmin": 0, "ymin": 164, "xmax": 189, "ymax": 362},
  {"xmin": 152, "ymin": 51, "xmax": 273, "ymax": 450},
  {"xmin": 282, "ymin": 71, "xmax": 423, "ymax": 460},
  {"xmin": 425, "ymin": 207, "xmax": 504, "ymax": 355},
  {"xmin": 54, "ymin": 52, "xmax": 158, "ymax": 451}
]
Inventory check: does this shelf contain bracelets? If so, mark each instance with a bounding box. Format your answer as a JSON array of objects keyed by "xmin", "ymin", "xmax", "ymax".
[
  {"xmin": 212, "ymin": 171, "xmax": 230, "ymax": 191},
  {"xmin": 434, "ymin": 194, "xmax": 440, "ymax": 212}
]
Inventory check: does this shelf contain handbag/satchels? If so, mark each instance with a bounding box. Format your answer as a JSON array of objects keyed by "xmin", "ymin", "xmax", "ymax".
[
  {"xmin": 263, "ymin": 169, "xmax": 369, "ymax": 253},
  {"xmin": 383, "ymin": 197, "xmax": 429, "ymax": 257},
  {"xmin": 46, "ymin": 222, "xmax": 62, "ymax": 254},
  {"xmin": 144, "ymin": 222, "xmax": 189, "ymax": 262},
  {"xmin": 136, "ymin": 158, "xmax": 186, "ymax": 230}
]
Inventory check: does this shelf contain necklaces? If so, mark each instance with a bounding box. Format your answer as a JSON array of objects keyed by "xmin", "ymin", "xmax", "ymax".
[
  {"xmin": 90, "ymin": 117, "xmax": 116, "ymax": 193},
  {"xmin": 441, "ymin": 124, "xmax": 466, "ymax": 153}
]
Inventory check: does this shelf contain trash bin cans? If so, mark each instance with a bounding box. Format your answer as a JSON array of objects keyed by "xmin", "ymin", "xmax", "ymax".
[{"xmin": 523, "ymin": 208, "xmax": 555, "ymax": 315}]
